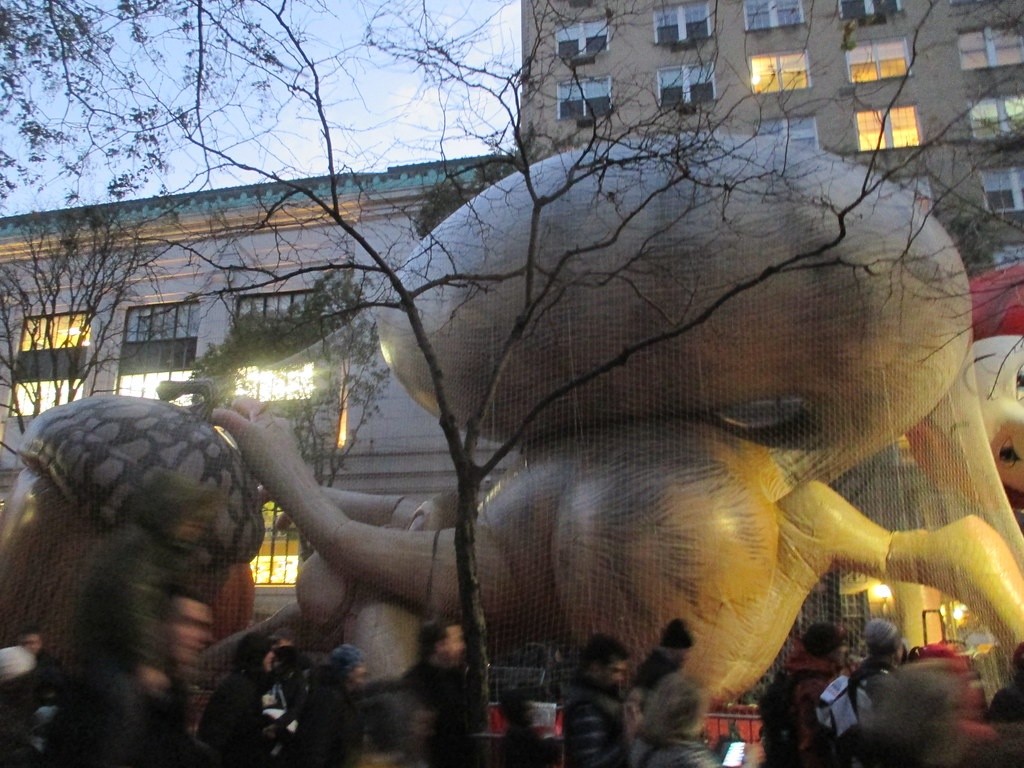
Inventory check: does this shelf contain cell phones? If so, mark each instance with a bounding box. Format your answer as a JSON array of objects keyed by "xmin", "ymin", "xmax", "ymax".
[{"xmin": 721, "ymin": 741, "xmax": 746, "ymax": 768}]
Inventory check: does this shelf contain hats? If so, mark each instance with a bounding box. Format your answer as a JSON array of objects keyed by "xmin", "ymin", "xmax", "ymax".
[
  {"xmin": 864, "ymin": 618, "xmax": 902, "ymax": 656},
  {"xmin": 325, "ymin": 643, "xmax": 367, "ymax": 673},
  {"xmin": 803, "ymin": 621, "xmax": 848, "ymax": 656},
  {"xmin": 1, "ymin": 646, "xmax": 39, "ymax": 685},
  {"xmin": 659, "ymin": 617, "xmax": 699, "ymax": 648}
]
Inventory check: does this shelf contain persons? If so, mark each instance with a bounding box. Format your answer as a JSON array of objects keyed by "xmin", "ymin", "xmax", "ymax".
[{"xmin": 0, "ymin": 464, "xmax": 1024, "ymax": 768}]
